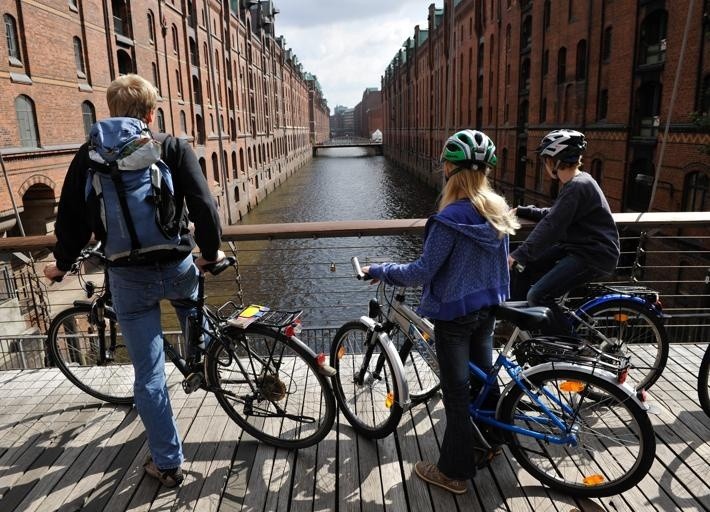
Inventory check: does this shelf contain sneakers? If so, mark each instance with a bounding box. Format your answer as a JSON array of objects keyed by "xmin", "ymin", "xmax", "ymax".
[
  {"xmin": 415, "ymin": 460, "xmax": 468, "ymax": 494},
  {"xmin": 142, "ymin": 453, "xmax": 184, "ymax": 488}
]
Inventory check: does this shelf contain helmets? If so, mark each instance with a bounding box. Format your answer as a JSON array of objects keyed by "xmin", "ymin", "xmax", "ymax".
[
  {"xmin": 534, "ymin": 129, "xmax": 587, "ymax": 159},
  {"xmin": 439, "ymin": 129, "xmax": 499, "ymax": 170}
]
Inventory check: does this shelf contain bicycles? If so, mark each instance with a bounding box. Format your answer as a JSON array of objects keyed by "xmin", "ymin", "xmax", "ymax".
[
  {"xmin": 330, "ymin": 257, "xmax": 656, "ymax": 498},
  {"xmin": 697, "ymin": 344, "xmax": 709, "ymax": 420},
  {"xmin": 383, "ymin": 211, "xmax": 669, "ymax": 406},
  {"xmin": 46, "ymin": 241, "xmax": 336, "ymax": 449}
]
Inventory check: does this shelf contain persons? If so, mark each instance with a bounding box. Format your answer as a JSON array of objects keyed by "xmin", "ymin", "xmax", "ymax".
[
  {"xmin": 506, "ymin": 129, "xmax": 621, "ymax": 376},
  {"xmin": 43, "ymin": 72, "xmax": 226, "ymax": 487},
  {"xmin": 361, "ymin": 130, "xmax": 511, "ymax": 495}
]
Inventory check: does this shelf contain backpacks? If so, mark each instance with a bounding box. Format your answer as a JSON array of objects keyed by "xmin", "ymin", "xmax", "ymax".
[{"xmin": 84, "ymin": 118, "xmax": 181, "ymax": 263}]
